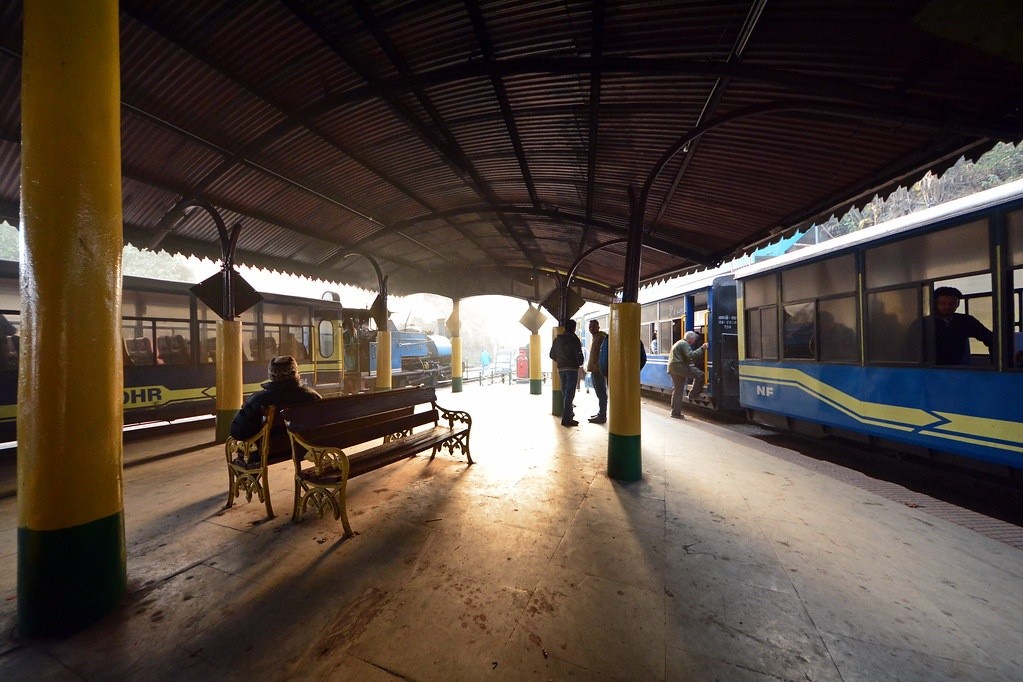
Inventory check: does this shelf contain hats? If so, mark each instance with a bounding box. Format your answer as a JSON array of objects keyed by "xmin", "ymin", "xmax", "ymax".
[{"xmin": 268, "ymin": 355, "xmax": 298, "ymax": 382}]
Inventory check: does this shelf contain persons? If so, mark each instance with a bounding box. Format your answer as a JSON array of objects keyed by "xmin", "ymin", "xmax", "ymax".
[
  {"xmin": 0, "ymin": 314, "xmax": 17, "ymax": 368},
  {"xmin": 480, "ymin": 348, "xmax": 492, "ymax": 374},
  {"xmin": 587, "ymin": 319, "xmax": 608, "ymax": 423},
  {"xmin": 230, "ymin": 355, "xmax": 323, "ymax": 484},
  {"xmin": 651, "ymin": 330, "xmax": 657, "ymax": 354},
  {"xmin": 550, "ymin": 320, "xmax": 584, "ymax": 426},
  {"xmin": 600, "ymin": 332, "xmax": 647, "ymax": 377},
  {"xmin": 667, "ymin": 331, "xmax": 708, "ymax": 418},
  {"xmin": 907, "ymin": 287, "xmax": 992, "ymax": 364},
  {"xmin": 674, "ymin": 321, "xmax": 680, "ymax": 340}
]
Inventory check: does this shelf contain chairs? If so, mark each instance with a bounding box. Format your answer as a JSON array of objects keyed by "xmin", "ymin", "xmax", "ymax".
[
  {"xmin": 0, "ymin": 315, "xmax": 19, "ymax": 371},
  {"xmin": 123, "ymin": 333, "xmax": 305, "ymax": 365},
  {"xmin": 781, "ymin": 323, "xmax": 1023, "ymax": 371}
]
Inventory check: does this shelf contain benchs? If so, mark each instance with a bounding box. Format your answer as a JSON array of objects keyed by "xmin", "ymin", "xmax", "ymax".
[
  {"xmin": 283, "ymin": 387, "xmax": 473, "ymax": 534},
  {"xmin": 226, "ymin": 385, "xmax": 417, "ymax": 518}
]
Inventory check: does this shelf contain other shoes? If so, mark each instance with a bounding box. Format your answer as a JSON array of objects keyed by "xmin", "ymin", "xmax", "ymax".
[
  {"xmin": 690, "ymin": 395, "xmax": 703, "ymax": 400},
  {"xmin": 561, "ymin": 419, "xmax": 579, "ymax": 426},
  {"xmin": 588, "ymin": 415, "xmax": 607, "ymax": 423},
  {"xmin": 671, "ymin": 414, "xmax": 684, "ymax": 419}
]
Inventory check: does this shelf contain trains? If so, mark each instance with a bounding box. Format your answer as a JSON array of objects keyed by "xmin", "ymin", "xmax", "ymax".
[
  {"xmin": 573, "ymin": 179, "xmax": 1023, "ymax": 489},
  {"xmin": 0, "ymin": 259, "xmax": 466, "ymax": 446}
]
